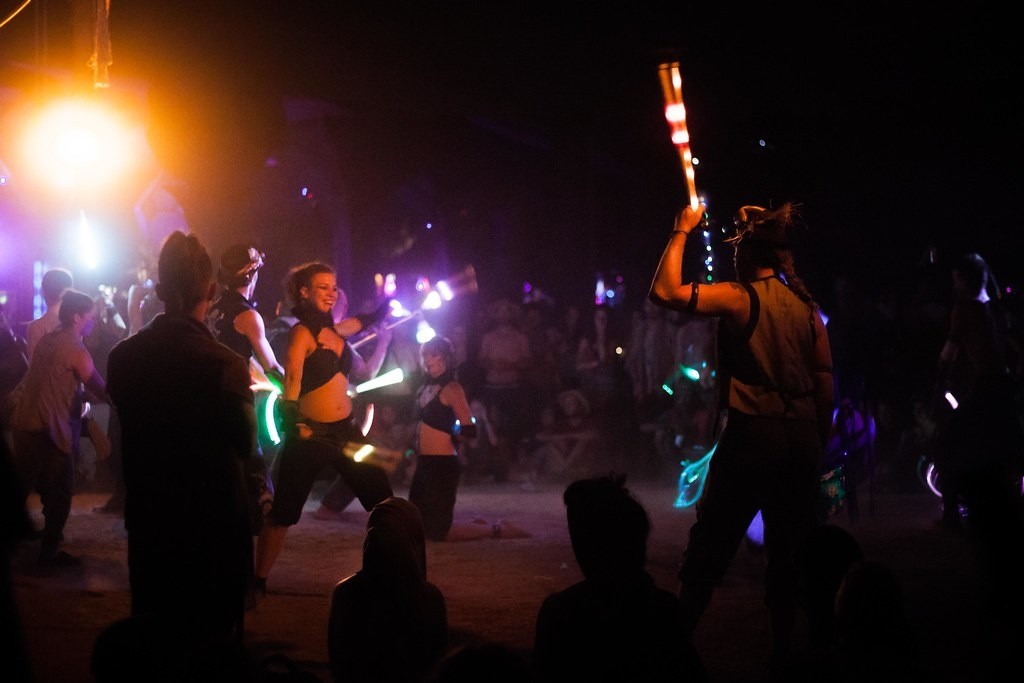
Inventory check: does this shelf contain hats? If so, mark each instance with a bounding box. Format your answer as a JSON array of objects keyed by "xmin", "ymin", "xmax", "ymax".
[
  {"xmin": 723, "ymin": 200, "xmax": 801, "ymax": 246},
  {"xmin": 219, "ymin": 243, "xmax": 264, "ymax": 279}
]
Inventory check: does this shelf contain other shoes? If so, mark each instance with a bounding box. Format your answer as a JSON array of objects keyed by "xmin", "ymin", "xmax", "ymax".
[
  {"xmin": 90, "ymin": 504, "xmax": 125, "ymax": 518},
  {"xmin": 244, "ymin": 587, "xmax": 267, "ymax": 610},
  {"xmin": 305, "ymin": 508, "xmax": 343, "ymax": 520}
]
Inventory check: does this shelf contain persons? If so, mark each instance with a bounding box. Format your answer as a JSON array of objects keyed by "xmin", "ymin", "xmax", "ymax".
[
  {"xmin": 326, "ymin": 497, "xmax": 450, "ymax": 683},
  {"xmin": 2, "ymin": 229, "xmax": 1018, "ymax": 513},
  {"xmin": 1, "ymin": 419, "xmax": 44, "ymax": 681},
  {"xmin": 439, "ymin": 643, "xmax": 539, "ymax": 682},
  {"xmin": 88, "ymin": 617, "xmax": 321, "ymax": 683},
  {"xmin": 244, "ymin": 261, "xmax": 396, "ymax": 612},
  {"xmin": 535, "ymin": 476, "xmax": 713, "ymax": 681},
  {"xmin": 648, "ymin": 201, "xmax": 835, "ymax": 682},
  {"xmin": 105, "ymin": 238, "xmax": 256, "ymax": 645},
  {"xmin": 790, "ymin": 525, "xmax": 924, "ymax": 683},
  {"xmin": 406, "ymin": 338, "xmax": 532, "ymax": 545}
]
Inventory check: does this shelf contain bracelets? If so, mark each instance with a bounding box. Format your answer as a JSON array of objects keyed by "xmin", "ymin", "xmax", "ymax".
[{"xmin": 668, "ymin": 229, "xmax": 688, "ymax": 237}]
